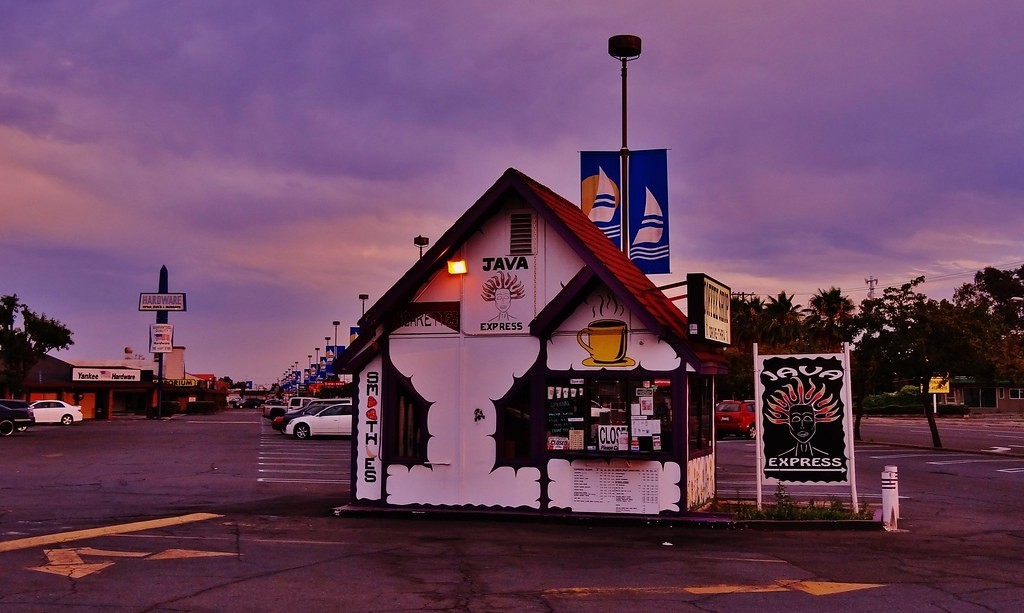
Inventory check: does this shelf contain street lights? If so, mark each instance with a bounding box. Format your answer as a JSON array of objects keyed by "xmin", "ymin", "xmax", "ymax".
[
  {"xmin": 607, "ymin": 32, "xmax": 642, "ymax": 255},
  {"xmin": 358, "ymin": 294, "xmax": 369, "ymax": 317},
  {"xmin": 283, "ymin": 348, "xmax": 321, "ymax": 396},
  {"xmin": 325, "ymin": 337, "xmax": 331, "ymax": 383},
  {"xmin": 332, "ymin": 320, "xmax": 340, "ymax": 362},
  {"xmin": 414, "ymin": 235, "xmax": 428, "ymax": 259}
]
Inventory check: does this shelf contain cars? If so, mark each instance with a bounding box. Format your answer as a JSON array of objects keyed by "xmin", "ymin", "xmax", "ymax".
[
  {"xmin": 0, "ymin": 399, "xmax": 35, "ymax": 435},
  {"xmin": 714, "ymin": 401, "xmax": 756, "ymax": 437},
  {"xmin": 261, "ymin": 398, "xmax": 353, "ymax": 431},
  {"xmin": 30, "ymin": 400, "xmax": 83, "ymax": 426},
  {"xmin": 233, "ymin": 399, "xmax": 261, "ymax": 409},
  {"xmin": 281, "ymin": 403, "xmax": 352, "ymax": 439}
]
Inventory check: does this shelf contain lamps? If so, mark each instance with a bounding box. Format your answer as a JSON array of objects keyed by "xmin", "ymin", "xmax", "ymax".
[{"xmin": 447, "ymin": 258, "xmax": 469, "ymax": 275}]
